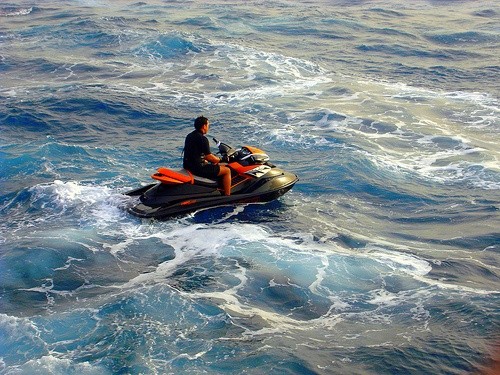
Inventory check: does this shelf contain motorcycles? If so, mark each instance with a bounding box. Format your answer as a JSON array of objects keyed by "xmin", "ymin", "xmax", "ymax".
[{"xmin": 123, "ymin": 137, "xmax": 300, "ymax": 218}]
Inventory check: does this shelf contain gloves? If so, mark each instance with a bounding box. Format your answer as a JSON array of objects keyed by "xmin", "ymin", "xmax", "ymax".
[{"xmin": 219, "ymin": 154, "xmax": 228, "ymax": 162}]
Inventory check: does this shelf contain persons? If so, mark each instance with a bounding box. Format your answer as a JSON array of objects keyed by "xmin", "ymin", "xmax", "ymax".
[{"xmin": 182, "ymin": 117, "xmax": 230, "ymax": 196}]
